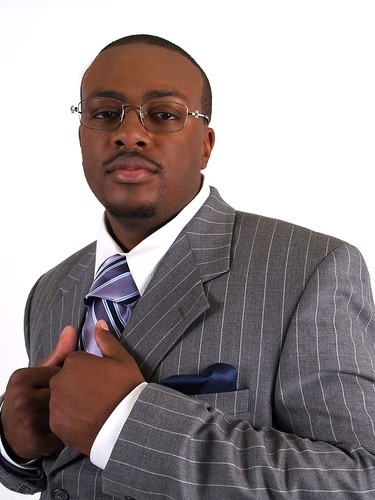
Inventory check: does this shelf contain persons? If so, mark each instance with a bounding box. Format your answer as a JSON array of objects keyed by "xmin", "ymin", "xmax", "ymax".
[{"xmin": 0, "ymin": 34, "xmax": 375, "ymax": 500}]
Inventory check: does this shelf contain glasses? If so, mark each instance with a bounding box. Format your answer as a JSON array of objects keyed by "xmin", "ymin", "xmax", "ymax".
[{"xmin": 71, "ymin": 98, "xmax": 210, "ymax": 134}]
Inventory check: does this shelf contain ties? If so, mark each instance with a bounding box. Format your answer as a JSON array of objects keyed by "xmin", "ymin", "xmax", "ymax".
[{"xmin": 79, "ymin": 255, "xmax": 140, "ymax": 356}]
